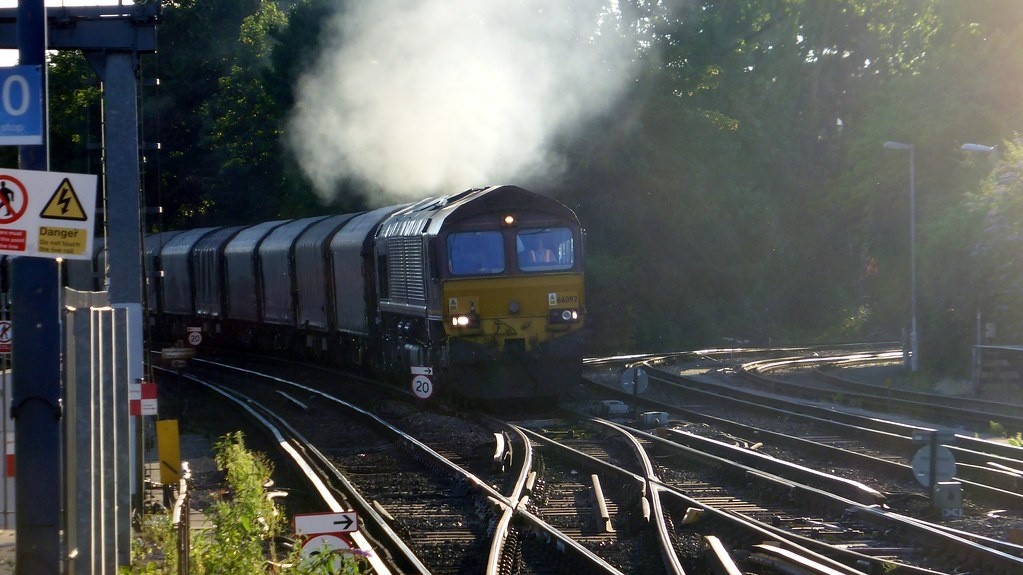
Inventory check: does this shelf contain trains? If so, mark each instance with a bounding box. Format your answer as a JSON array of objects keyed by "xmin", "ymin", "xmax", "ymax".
[{"xmin": 77, "ymin": 180, "xmax": 589, "ymax": 395}]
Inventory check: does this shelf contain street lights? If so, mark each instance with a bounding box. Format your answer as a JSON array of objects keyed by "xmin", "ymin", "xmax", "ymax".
[
  {"xmin": 881, "ymin": 138, "xmax": 923, "ymax": 373},
  {"xmin": 963, "ymin": 143, "xmax": 998, "ymax": 380}
]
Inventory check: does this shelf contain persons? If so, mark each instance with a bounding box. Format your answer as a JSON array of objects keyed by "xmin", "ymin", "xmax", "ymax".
[{"xmin": 527, "ymin": 237, "xmax": 557, "ymax": 267}]
